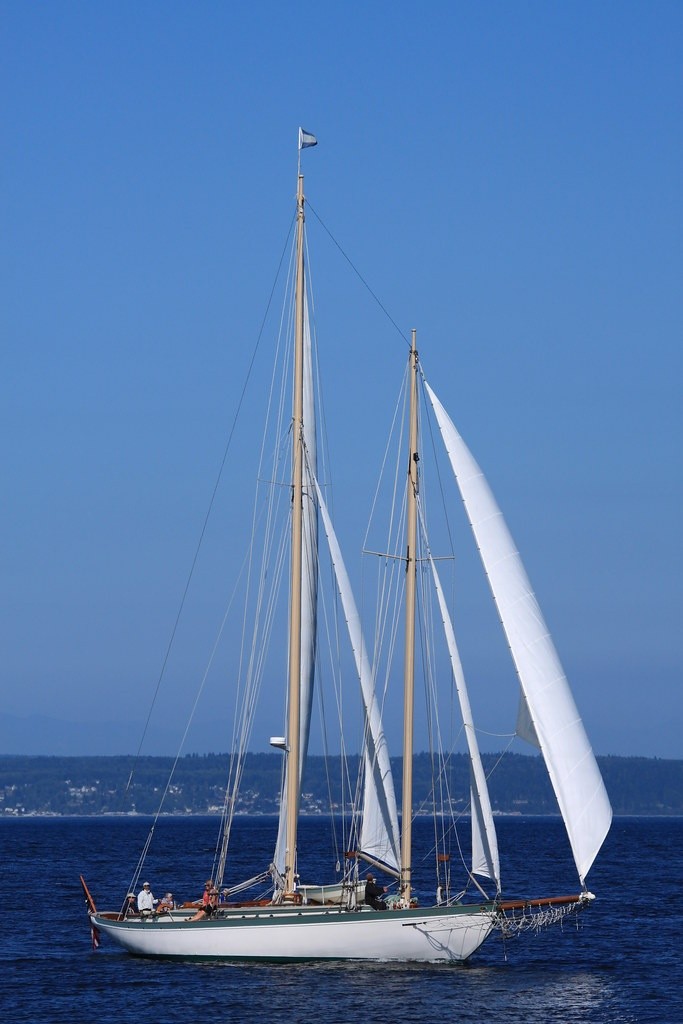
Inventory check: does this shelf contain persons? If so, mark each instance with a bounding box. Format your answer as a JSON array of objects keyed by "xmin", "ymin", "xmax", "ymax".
[
  {"xmin": 184, "ymin": 880, "xmax": 219, "ymax": 921},
  {"xmin": 161, "ymin": 893, "xmax": 173, "ymax": 909},
  {"xmin": 124, "ymin": 893, "xmax": 139, "ymax": 913},
  {"xmin": 137, "ymin": 882, "xmax": 161, "ymax": 912},
  {"xmin": 365, "ymin": 873, "xmax": 388, "ymax": 910}
]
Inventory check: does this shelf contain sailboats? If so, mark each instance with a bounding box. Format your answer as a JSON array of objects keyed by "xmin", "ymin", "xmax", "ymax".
[{"xmin": 77, "ymin": 126, "xmax": 612, "ymax": 965}]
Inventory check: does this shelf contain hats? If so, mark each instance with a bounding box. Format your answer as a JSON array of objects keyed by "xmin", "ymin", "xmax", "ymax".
[
  {"xmin": 367, "ymin": 873, "xmax": 373, "ymax": 880},
  {"xmin": 127, "ymin": 893, "xmax": 136, "ymax": 897},
  {"xmin": 143, "ymin": 882, "xmax": 150, "ymax": 885}
]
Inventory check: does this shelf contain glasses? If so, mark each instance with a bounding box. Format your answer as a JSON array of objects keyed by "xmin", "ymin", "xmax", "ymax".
[
  {"xmin": 208, "ymin": 894, "xmax": 216, "ymax": 897},
  {"xmin": 205, "ymin": 884, "xmax": 211, "ymax": 886}
]
